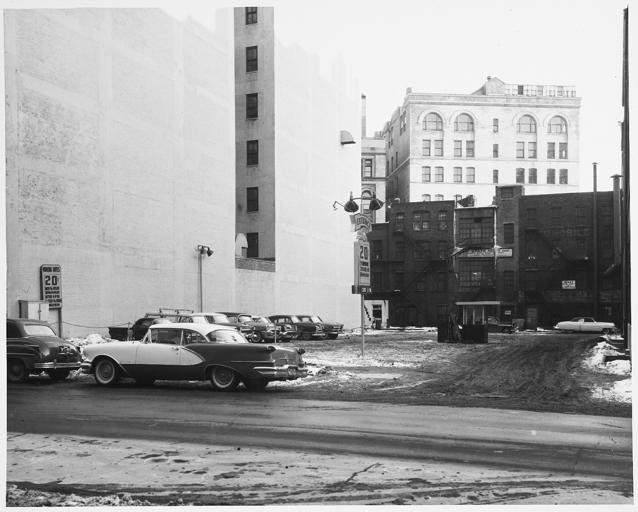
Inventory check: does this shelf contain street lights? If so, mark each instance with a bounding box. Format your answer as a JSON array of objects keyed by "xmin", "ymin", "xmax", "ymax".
[
  {"xmin": 198, "ymin": 245, "xmax": 214, "ymax": 314},
  {"xmin": 344, "ymin": 190, "xmax": 384, "ymax": 358}
]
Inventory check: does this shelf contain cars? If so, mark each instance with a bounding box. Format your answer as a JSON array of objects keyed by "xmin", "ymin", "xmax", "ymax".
[
  {"xmin": 7, "ymin": 319, "xmax": 81, "ymax": 381},
  {"xmin": 82, "ymin": 323, "xmax": 313, "ymax": 392},
  {"xmin": 109, "ymin": 308, "xmax": 344, "ymax": 344}
]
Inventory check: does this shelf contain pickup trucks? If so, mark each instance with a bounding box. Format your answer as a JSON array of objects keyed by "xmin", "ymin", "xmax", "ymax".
[{"xmin": 554, "ymin": 317, "xmax": 615, "ymax": 333}]
